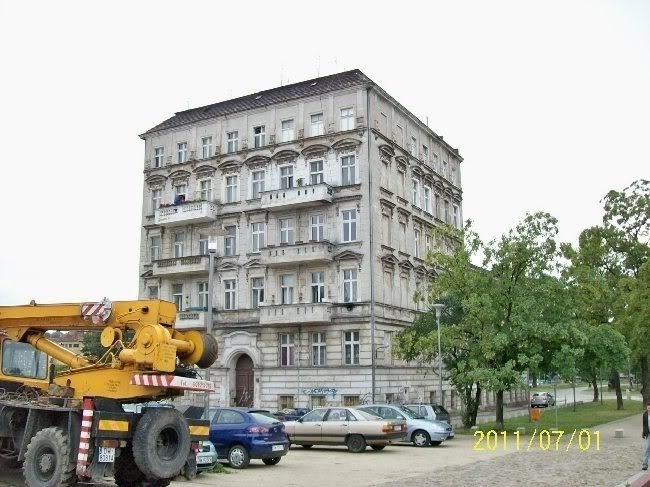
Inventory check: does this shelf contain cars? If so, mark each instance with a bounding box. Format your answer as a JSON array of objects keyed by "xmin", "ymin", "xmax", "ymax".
[
  {"xmin": 406, "ymin": 402, "xmax": 450, "ymax": 423},
  {"xmin": 207, "ymin": 407, "xmax": 291, "ymax": 469},
  {"xmin": 274, "ymin": 408, "xmax": 312, "ymax": 421},
  {"xmin": 282, "ymin": 408, "xmax": 408, "ymax": 452},
  {"xmin": 531, "ymin": 392, "xmax": 555, "ymax": 408},
  {"xmin": 353, "ymin": 404, "xmax": 452, "ymax": 447}
]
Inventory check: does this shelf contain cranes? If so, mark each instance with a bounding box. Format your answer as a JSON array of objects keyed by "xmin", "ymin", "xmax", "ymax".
[{"xmin": 0, "ymin": 299, "xmax": 218, "ymax": 487}]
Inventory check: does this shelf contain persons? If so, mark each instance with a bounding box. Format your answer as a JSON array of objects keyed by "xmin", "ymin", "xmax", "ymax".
[{"xmin": 641, "ymin": 402, "xmax": 650, "ymax": 470}]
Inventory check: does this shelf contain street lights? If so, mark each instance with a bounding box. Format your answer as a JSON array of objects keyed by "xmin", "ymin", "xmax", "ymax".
[
  {"xmin": 431, "ymin": 304, "xmax": 446, "ymax": 408},
  {"xmin": 366, "ymin": 83, "xmax": 384, "ymax": 404},
  {"xmin": 204, "ymin": 236, "xmax": 217, "ymax": 421}
]
